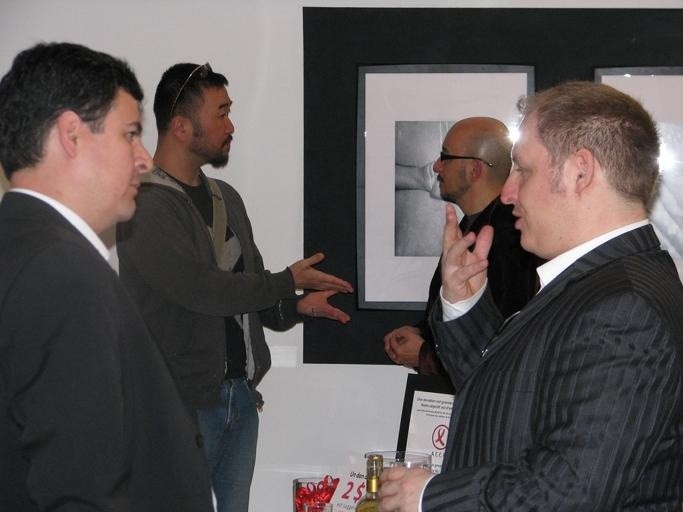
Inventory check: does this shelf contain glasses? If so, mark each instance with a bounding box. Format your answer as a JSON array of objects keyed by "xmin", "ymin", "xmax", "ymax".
[
  {"xmin": 171, "ymin": 62, "xmax": 211, "ymax": 122},
  {"xmin": 439, "ymin": 151, "xmax": 493, "ymax": 167}
]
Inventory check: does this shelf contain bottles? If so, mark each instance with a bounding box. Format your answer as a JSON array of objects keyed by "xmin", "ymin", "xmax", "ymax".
[{"xmin": 355, "ymin": 454, "xmax": 388, "ymax": 512}]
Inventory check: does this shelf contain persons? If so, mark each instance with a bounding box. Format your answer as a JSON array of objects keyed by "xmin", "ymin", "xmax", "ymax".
[
  {"xmin": 113, "ymin": 62, "xmax": 355, "ymax": 511},
  {"xmin": 374, "ymin": 81, "xmax": 682, "ymax": 511},
  {"xmin": 0, "ymin": 38, "xmax": 219, "ymax": 511},
  {"xmin": 383, "ymin": 117, "xmax": 542, "ymax": 374}
]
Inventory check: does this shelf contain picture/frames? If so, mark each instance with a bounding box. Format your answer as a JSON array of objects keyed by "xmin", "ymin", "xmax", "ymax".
[
  {"xmin": 357, "ymin": 64, "xmax": 536, "ymax": 311},
  {"xmin": 395, "ymin": 373, "xmax": 456, "ymax": 476},
  {"xmin": 594, "ymin": 66, "xmax": 683, "ymax": 287}
]
